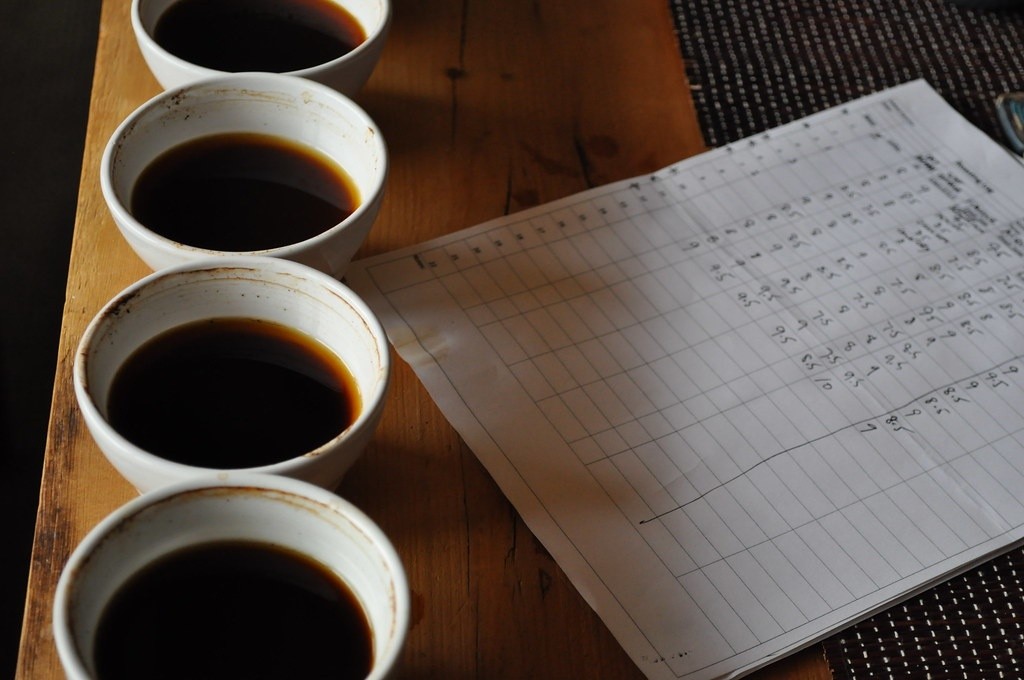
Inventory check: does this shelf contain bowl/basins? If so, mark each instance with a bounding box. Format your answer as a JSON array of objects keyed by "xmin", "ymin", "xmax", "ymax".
[
  {"xmin": 99, "ymin": 71, "xmax": 391, "ymax": 286},
  {"xmin": 129, "ymin": 0, "xmax": 391, "ymax": 103},
  {"xmin": 52, "ymin": 473, "xmax": 412, "ymax": 680},
  {"xmin": 71, "ymin": 253, "xmax": 395, "ymax": 498}
]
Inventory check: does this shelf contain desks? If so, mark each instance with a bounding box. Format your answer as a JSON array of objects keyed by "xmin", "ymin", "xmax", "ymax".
[{"xmin": 15, "ymin": 0, "xmax": 831, "ymax": 680}]
